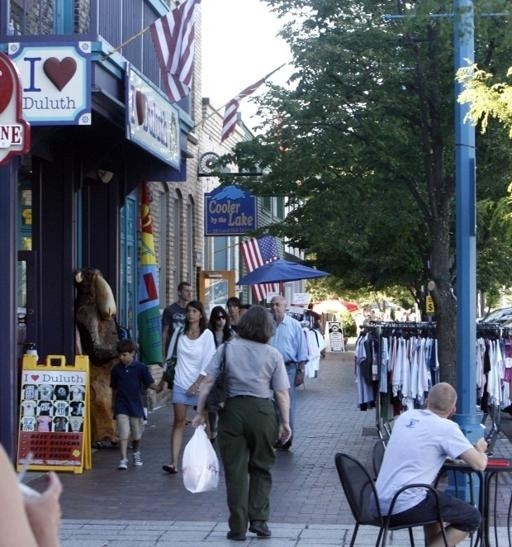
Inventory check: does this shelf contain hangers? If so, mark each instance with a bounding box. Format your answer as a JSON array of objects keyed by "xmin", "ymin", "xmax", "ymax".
[{"xmin": 353, "ymin": 315, "xmax": 507, "ymax": 340}]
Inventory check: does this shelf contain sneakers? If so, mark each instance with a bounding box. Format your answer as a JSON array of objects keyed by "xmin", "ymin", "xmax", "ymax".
[
  {"xmin": 118, "ymin": 460, "xmax": 128, "ymax": 469},
  {"xmin": 227, "ymin": 531, "xmax": 246, "ymax": 540},
  {"xmin": 133, "ymin": 453, "xmax": 143, "ymax": 465},
  {"xmin": 249, "ymin": 520, "xmax": 271, "ymax": 536},
  {"xmin": 273, "ymin": 436, "xmax": 292, "ymax": 450}
]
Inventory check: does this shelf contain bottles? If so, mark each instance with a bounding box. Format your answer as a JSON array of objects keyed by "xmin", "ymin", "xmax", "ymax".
[{"xmin": 24, "ymin": 340, "xmax": 39, "ymax": 362}]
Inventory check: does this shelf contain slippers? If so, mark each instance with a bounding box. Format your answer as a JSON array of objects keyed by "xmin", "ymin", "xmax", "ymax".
[{"xmin": 162, "ymin": 464, "xmax": 178, "ymax": 473}]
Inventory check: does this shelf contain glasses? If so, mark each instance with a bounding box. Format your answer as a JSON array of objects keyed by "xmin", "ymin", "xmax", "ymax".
[{"xmin": 217, "ymin": 316, "xmax": 225, "ymax": 320}]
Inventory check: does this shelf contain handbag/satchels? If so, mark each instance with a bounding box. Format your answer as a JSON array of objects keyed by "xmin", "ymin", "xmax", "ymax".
[
  {"xmin": 163, "ymin": 358, "xmax": 177, "ymax": 390},
  {"xmin": 205, "ymin": 372, "xmax": 229, "ymax": 412}
]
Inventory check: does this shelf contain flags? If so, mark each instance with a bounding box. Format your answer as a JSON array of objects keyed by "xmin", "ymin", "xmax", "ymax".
[
  {"xmin": 144, "ymin": 0, "xmax": 203, "ymax": 105},
  {"xmin": 218, "ymin": 73, "xmax": 271, "ymax": 146},
  {"xmin": 239, "ymin": 232, "xmax": 286, "ymax": 302}
]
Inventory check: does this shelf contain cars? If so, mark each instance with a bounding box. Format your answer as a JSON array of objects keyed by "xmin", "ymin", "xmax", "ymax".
[{"xmin": 476, "ymin": 305, "xmax": 512, "ymax": 337}]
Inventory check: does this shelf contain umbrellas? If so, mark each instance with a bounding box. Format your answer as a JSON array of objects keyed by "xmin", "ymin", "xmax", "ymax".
[
  {"xmin": 233, "ymin": 254, "xmax": 330, "ymax": 295},
  {"xmin": 312, "ymin": 298, "xmax": 359, "ymax": 322}
]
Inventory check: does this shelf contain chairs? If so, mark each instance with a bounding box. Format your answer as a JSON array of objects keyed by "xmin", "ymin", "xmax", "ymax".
[{"xmin": 333, "ymin": 383, "xmax": 510, "ymax": 546}]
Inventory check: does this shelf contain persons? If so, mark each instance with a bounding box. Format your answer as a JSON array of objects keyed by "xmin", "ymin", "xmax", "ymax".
[
  {"xmin": 156, "ymin": 279, "xmax": 323, "ymax": 472},
  {"xmin": 109, "ymin": 336, "xmax": 162, "ymax": 469},
  {"xmin": 0, "ymin": 442, "xmax": 64, "ymax": 546},
  {"xmin": 368, "ymin": 381, "xmax": 488, "ymax": 546},
  {"xmin": 188, "ymin": 304, "xmax": 295, "ymax": 541}
]
